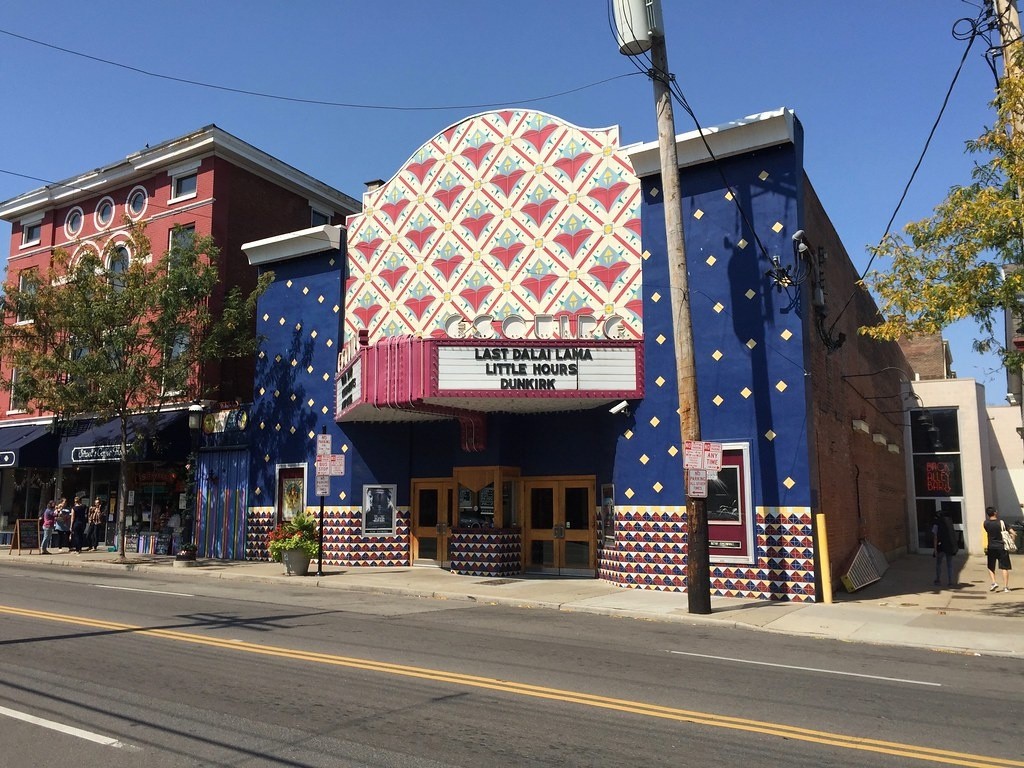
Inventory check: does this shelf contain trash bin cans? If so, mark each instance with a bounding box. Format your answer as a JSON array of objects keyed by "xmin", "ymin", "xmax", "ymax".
[{"xmin": 1008, "ymin": 521, "xmax": 1024, "ymax": 555}]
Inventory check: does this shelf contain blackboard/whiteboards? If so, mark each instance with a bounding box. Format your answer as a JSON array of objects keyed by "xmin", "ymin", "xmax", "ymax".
[{"xmin": 8, "ymin": 517, "xmax": 42, "ymax": 557}]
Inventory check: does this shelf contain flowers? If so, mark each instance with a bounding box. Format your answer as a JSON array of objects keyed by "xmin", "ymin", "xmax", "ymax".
[{"xmin": 266, "ymin": 510, "xmax": 324, "ymax": 561}]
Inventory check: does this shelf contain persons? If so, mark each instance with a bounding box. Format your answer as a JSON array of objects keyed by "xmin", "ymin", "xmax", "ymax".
[
  {"xmin": 932, "ymin": 511, "xmax": 959, "ymax": 586},
  {"xmin": 40, "ymin": 497, "xmax": 105, "ymax": 554},
  {"xmin": 984, "ymin": 507, "xmax": 1016, "ymax": 593}
]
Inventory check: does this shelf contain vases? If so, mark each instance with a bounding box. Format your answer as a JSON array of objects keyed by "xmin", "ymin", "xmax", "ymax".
[{"xmin": 279, "ymin": 549, "xmax": 310, "ymax": 576}]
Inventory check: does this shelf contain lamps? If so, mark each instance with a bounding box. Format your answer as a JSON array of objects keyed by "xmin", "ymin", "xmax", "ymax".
[
  {"xmin": 840, "ymin": 366, "xmax": 945, "ymax": 448},
  {"xmin": 188, "ymin": 396, "xmax": 204, "ymax": 411}
]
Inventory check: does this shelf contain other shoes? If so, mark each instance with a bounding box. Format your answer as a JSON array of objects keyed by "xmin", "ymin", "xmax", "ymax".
[{"xmin": 42, "ymin": 550, "xmax": 51, "ymax": 554}]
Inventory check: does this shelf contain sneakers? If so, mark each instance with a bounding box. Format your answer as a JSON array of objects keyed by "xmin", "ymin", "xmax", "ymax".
[
  {"xmin": 988, "ymin": 583, "xmax": 999, "ymax": 592},
  {"xmin": 1005, "ymin": 587, "xmax": 1013, "ymax": 592}
]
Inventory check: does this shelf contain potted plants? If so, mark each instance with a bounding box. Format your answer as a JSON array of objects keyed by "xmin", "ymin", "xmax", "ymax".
[{"xmin": 178, "ymin": 541, "xmax": 198, "ymax": 556}]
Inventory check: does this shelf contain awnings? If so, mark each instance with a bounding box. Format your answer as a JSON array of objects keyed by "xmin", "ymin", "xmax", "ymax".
[
  {"xmin": 58, "ymin": 411, "xmax": 191, "ymax": 466},
  {"xmin": 0, "ymin": 425, "xmax": 53, "ymax": 468}
]
